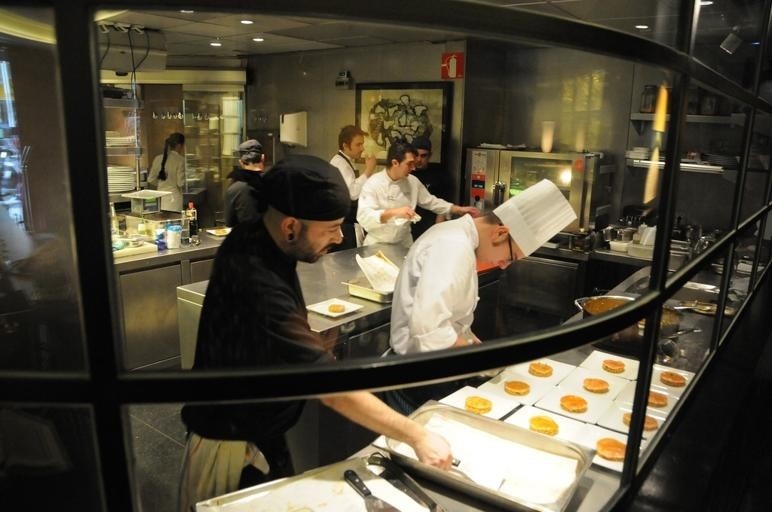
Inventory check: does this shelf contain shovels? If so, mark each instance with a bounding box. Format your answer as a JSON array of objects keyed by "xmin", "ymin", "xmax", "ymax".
[
  {"xmin": 344, "ymin": 470, "xmax": 403, "ymax": 512},
  {"xmin": 452, "ymin": 457, "xmax": 506, "ymax": 492}
]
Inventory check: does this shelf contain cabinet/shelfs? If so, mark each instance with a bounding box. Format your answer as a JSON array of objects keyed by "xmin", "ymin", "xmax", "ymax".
[
  {"xmin": 102, "ymin": 90, "xmax": 143, "ymax": 207},
  {"xmin": 624, "ymin": 111, "xmax": 771, "ymax": 180},
  {"xmin": 191, "ymin": 249, "xmax": 217, "ymax": 286},
  {"xmin": 118, "ymin": 254, "xmax": 182, "ymax": 376}
]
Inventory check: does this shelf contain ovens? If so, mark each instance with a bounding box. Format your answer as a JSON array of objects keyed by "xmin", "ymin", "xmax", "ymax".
[{"xmin": 461, "ymin": 147, "xmax": 615, "ymax": 249}]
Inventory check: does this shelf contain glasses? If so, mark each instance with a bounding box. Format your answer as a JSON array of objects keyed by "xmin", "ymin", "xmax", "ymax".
[{"xmin": 497, "ymin": 222, "xmax": 518, "ymax": 265}]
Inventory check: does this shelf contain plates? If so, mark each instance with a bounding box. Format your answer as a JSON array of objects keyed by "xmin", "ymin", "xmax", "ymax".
[
  {"xmin": 205, "ymin": 227, "xmax": 232, "ymax": 237},
  {"xmin": 435, "ymin": 350, "xmax": 699, "ymax": 473},
  {"xmin": 734, "ymin": 262, "xmax": 764, "ymax": 276},
  {"xmin": 621, "ymin": 146, "xmax": 739, "ymax": 167},
  {"xmin": 120, "ymin": 188, "xmax": 174, "ymax": 200},
  {"xmin": 107, "ymin": 163, "xmax": 137, "ymax": 193},
  {"xmin": 306, "ymin": 297, "xmax": 364, "ymax": 319},
  {"xmin": 105, "ymin": 130, "xmax": 137, "ymax": 148}
]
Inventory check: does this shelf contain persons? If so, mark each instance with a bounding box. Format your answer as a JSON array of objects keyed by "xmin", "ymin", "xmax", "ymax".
[
  {"xmin": 383, "ymin": 178, "xmax": 578, "ymax": 417},
  {"xmin": 178, "ymin": 155, "xmax": 453, "ymax": 512},
  {"xmin": 407, "ymin": 135, "xmax": 450, "ymax": 244},
  {"xmin": 223, "ymin": 139, "xmax": 267, "ymax": 229},
  {"xmin": 355, "ymin": 141, "xmax": 481, "ymax": 252},
  {"xmin": 147, "ymin": 132, "xmax": 187, "ymax": 215},
  {"xmin": 328, "ymin": 123, "xmax": 377, "ymax": 252}
]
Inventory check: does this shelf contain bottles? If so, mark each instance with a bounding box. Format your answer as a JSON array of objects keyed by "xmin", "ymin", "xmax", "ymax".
[
  {"xmin": 180, "ymin": 201, "xmax": 199, "ymax": 244},
  {"xmin": 640, "ymin": 83, "xmax": 738, "ymax": 116},
  {"xmin": 608, "ymin": 215, "xmax": 657, "ymax": 247},
  {"xmin": 109, "ymin": 201, "xmax": 120, "ymax": 239},
  {"xmin": 137, "ymin": 213, "xmax": 182, "ymax": 250}
]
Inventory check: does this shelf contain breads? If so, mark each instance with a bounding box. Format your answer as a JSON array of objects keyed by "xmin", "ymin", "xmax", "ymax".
[
  {"xmin": 583, "ymin": 378, "xmax": 608, "ymax": 393},
  {"xmin": 623, "ymin": 413, "xmax": 632, "ymax": 426},
  {"xmin": 596, "ymin": 438, "xmax": 627, "ymax": 461},
  {"xmin": 660, "ymin": 372, "xmax": 686, "ymax": 387},
  {"xmin": 328, "ymin": 304, "xmax": 344, "ymax": 313},
  {"xmin": 466, "ymin": 396, "xmax": 492, "ymax": 414},
  {"xmin": 560, "ymin": 395, "xmax": 587, "ymax": 413},
  {"xmin": 602, "ymin": 359, "xmax": 625, "ymax": 373},
  {"xmin": 504, "ymin": 381, "xmax": 530, "ymax": 396},
  {"xmin": 529, "ymin": 416, "xmax": 559, "ymax": 436},
  {"xmin": 647, "ymin": 391, "xmax": 668, "ymax": 407},
  {"xmin": 643, "ymin": 416, "xmax": 657, "ymax": 430},
  {"xmin": 529, "ymin": 362, "xmax": 553, "ymax": 377}
]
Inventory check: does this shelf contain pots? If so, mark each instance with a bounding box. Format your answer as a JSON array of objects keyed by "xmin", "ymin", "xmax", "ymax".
[{"xmin": 574, "ymin": 295, "xmax": 703, "ymax": 350}]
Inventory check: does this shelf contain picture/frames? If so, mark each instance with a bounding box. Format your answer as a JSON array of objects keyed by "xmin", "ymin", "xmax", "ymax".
[{"xmin": 354, "ymin": 80, "xmax": 454, "ymax": 167}]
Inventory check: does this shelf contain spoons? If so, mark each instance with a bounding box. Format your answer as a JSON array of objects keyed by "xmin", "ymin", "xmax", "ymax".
[{"xmin": 673, "ymin": 304, "xmax": 712, "ymax": 312}]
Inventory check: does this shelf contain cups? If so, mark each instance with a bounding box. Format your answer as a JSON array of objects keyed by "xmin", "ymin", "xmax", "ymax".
[
  {"xmin": 540, "ymin": 119, "xmax": 555, "ymax": 152},
  {"xmin": 214, "ymin": 211, "xmax": 226, "ymax": 233}
]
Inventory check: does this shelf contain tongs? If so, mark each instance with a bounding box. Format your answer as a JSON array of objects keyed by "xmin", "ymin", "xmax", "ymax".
[{"xmin": 366, "ymin": 450, "xmax": 445, "ymax": 511}]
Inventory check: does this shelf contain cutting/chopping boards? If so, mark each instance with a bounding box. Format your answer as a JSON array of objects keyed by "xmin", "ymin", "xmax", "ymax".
[{"xmin": 111, "ymin": 237, "xmax": 158, "ymax": 258}]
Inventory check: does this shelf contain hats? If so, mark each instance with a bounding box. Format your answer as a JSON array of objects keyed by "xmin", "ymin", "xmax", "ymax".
[
  {"xmin": 489, "ymin": 177, "xmax": 578, "ymax": 258},
  {"xmin": 259, "ymin": 153, "xmax": 352, "ymax": 221},
  {"xmin": 237, "ymin": 139, "xmax": 264, "ymax": 162}
]
